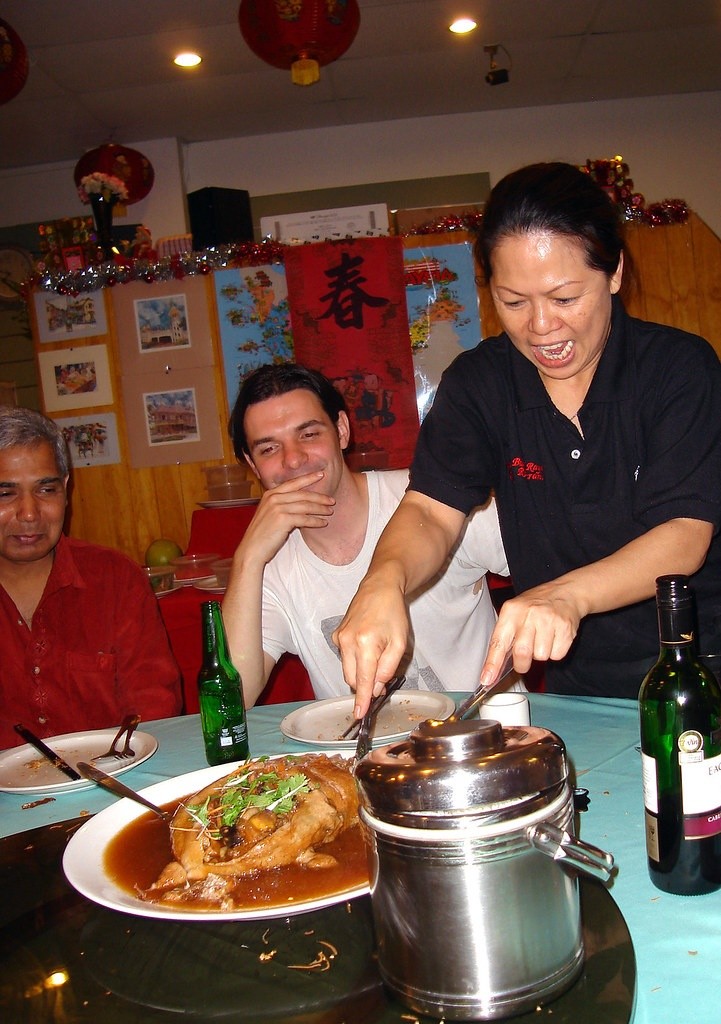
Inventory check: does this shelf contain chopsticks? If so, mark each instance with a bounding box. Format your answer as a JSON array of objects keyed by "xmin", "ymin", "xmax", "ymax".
[
  {"xmin": 13, "ymin": 723, "xmax": 81, "ymax": 781},
  {"xmin": 338, "ymin": 676, "xmax": 405, "ymax": 740}
]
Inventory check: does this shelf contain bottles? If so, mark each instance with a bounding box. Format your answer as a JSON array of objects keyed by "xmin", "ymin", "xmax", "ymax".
[
  {"xmin": 196, "ymin": 600, "xmax": 249, "ymax": 765},
  {"xmin": 637, "ymin": 574, "xmax": 721, "ymax": 897}
]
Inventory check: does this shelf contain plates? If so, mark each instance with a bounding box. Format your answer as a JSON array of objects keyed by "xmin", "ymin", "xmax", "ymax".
[
  {"xmin": 279, "ymin": 690, "xmax": 456, "ymax": 749},
  {"xmin": 0, "ymin": 730, "xmax": 158, "ymax": 794},
  {"xmin": 172, "ymin": 574, "xmax": 215, "ymax": 587},
  {"xmin": 192, "ymin": 577, "xmax": 228, "ymax": 594},
  {"xmin": 195, "ymin": 497, "xmax": 261, "ymax": 509},
  {"xmin": 62, "ymin": 749, "xmax": 370, "ymax": 921},
  {"xmin": 156, "ymin": 584, "xmax": 183, "ymax": 597}
]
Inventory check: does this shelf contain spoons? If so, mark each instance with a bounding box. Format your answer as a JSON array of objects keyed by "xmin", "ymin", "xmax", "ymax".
[{"xmin": 90, "ymin": 715, "xmax": 134, "ymax": 763}]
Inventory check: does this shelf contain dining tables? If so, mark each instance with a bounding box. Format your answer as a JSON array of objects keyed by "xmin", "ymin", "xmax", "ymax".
[{"xmin": 0, "ymin": 691, "xmax": 721, "ymax": 1023}]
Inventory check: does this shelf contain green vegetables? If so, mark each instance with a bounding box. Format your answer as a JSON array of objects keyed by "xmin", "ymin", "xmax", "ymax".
[{"xmin": 168, "ymin": 754, "xmax": 322, "ymax": 839}]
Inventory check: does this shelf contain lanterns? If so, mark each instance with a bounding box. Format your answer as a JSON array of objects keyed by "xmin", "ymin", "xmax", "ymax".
[
  {"xmin": 238, "ymin": 0, "xmax": 361, "ymax": 86},
  {"xmin": 0, "ymin": 18, "xmax": 28, "ymax": 107},
  {"xmin": 74, "ymin": 143, "xmax": 154, "ymax": 218}
]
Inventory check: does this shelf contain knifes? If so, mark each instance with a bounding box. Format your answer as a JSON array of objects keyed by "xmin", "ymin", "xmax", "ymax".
[{"xmin": 356, "ymin": 695, "xmax": 372, "ymax": 763}]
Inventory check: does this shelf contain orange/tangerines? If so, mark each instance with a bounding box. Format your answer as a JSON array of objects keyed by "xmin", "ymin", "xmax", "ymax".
[{"xmin": 145, "ymin": 539, "xmax": 184, "ymax": 569}]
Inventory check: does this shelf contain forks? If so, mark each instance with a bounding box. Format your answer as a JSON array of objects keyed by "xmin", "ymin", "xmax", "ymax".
[{"xmin": 114, "ymin": 715, "xmax": 141, "ymax": 761}]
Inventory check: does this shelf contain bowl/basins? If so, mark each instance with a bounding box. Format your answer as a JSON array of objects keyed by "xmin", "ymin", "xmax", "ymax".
[
  {"xmin": 203, "ymin": 479, "xmax": 254, "ymax": 500},
  {"xmin": 208, "ymin": 557, "xmax": 234, "ymax": 586},
  {"xmin": 143, "ymin": 566, "xmax": 177, "ymax": 592},
  {"xmin": 171, "ymin": 553, "xmax": 219, "ymax": 578},
  {"xmin": 347, "ymin": 450, "xmax": 391, "ymax": 471},
  {"xmin": 200, "ymin": 463, "xmax": 250, "ymax": 484}
]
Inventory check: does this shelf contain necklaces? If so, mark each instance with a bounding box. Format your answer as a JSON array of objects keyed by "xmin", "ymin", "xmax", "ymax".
[{"xmin": 568, "ymin": 414, "xmax": 579, "ymax": 422}]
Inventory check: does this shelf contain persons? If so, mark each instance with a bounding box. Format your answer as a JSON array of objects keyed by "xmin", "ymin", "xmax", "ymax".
[
  {"xmin": 0, "ymin": 405, "xmax": 186, "ymax": 760},
  {"xmin": 218, "ymin": 364, "xmax": 530, "ymax": 708},
  {"xmin": 333, "ymin": 164, "xmax": 721, "ymax": 727}
]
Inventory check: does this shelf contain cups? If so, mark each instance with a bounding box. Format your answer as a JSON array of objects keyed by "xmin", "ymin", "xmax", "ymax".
[{"xmin": 478, "ymin": 692, "xmax": 531, "ymax": 727}]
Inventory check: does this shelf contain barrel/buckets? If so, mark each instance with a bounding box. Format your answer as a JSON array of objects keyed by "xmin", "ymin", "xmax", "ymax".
[{"xmin": 351, "ymin": 715, "xmax": 616, "ymax": 1023}]
